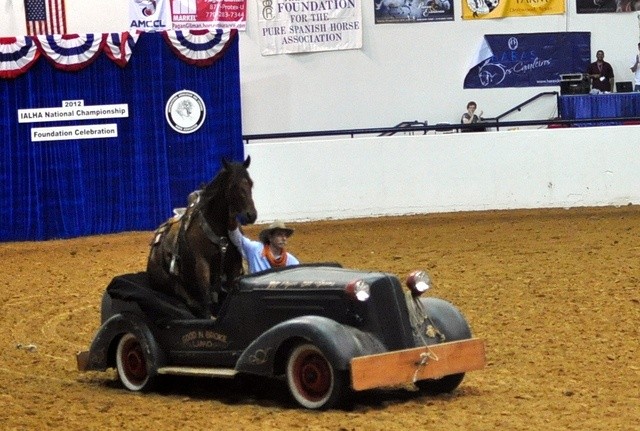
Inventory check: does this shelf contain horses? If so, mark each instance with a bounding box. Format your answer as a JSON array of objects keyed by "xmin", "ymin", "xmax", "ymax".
[{"xmin": 148, "ymin": 154, "xmax": 257, "ymax": 320}]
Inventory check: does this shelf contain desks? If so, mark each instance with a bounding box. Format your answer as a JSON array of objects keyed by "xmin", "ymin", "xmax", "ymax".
[{"xmin": 558, "ymin": 92, "xmax": 640, "ymax": 127}]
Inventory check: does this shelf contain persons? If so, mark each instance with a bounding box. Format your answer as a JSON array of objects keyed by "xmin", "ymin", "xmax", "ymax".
[
  {"xmin": 460, "ymin": 101, "xmax": 479, "ymax": 125},
  {"xmin": 587, "ymin": 50, "xmax": 614, "ymax": 93},
  {"xmin": 630, "ymin": 42, "xmax": 640, "ymax": 92},
  {"xmin": 226, "ymin": 203, "xmax": 300, "ymax": 274}
]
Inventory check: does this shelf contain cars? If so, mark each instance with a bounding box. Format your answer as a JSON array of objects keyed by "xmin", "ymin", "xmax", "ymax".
[{"xmin": 77, "ymin": 263, "xmax": 486, "ymax": 409}]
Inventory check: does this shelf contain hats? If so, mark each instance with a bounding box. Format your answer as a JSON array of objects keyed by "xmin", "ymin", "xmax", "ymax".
[{"xmin": 258, "ymin": 221, "xmax": 295, "ymax": 242}]
